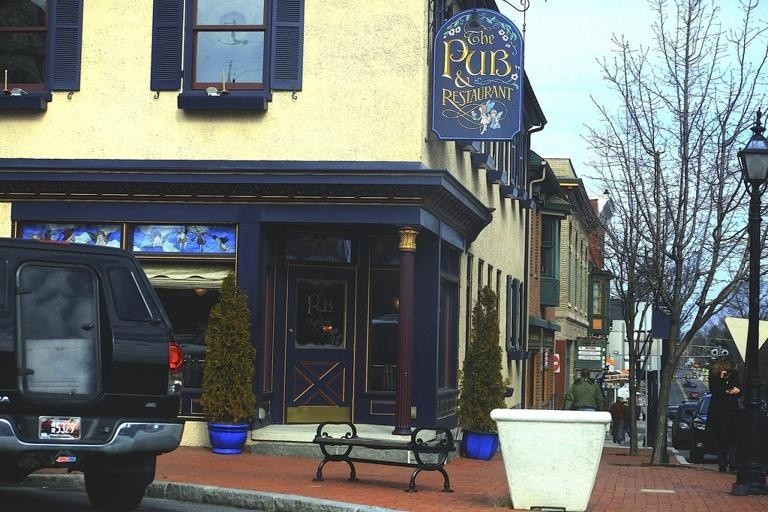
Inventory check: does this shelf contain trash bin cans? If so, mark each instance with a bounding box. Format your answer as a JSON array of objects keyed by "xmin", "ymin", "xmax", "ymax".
[{"xmin": 491, "ymin": 407, "xmax": 611, "ymax": 512}]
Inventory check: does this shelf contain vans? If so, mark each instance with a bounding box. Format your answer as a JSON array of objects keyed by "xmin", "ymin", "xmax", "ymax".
[{"xmin": 689, "ymin": 379, "xmax": 696, "ymax": 388}]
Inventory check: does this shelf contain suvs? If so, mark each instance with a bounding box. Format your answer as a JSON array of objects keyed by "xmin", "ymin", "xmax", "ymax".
[
  {"xmin": 1, "ymin": 238, "xmax": 185, "ymax": 510},
  {"xmin": 690, "ymin": 392, "xmax": 728, "ymax": 465},
  {"xmin": 671, "ymin": 401, "xmax": 699, "ymax": 450}
]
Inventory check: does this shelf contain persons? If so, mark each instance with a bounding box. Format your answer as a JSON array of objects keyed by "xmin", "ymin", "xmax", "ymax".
[
  {"xmin": 704, "ymin": 358, "xmax": 744, "ymax": 473},
  {"xmin": 478, "ymin": 100, "xmax": 495, "ymax": 134},
  {"xmin": 625, "ymin": 400, "xmax": 631, "ymax": 437},
  {"xmin": 563, "ymin": 368, "xmax": 603, "ymax": 412},
  {"xmin": 608, "ymin": 398, "xmax": 626, "ymax": 445},
  {"xmin": 489, "ymin": 110, "xmax": 503, "ymax": 130}
]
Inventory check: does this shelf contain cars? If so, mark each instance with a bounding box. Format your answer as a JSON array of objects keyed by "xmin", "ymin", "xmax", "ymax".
[
  {"xmin": 690, "ymin": 393, "xmax": 700, "ymax": 399},
  {"xmin": 683, "ymin": 382, "xmax": 688, "ymax": 387},
  {"xmin": 673, "ymin": 371, "xmax": 678, "ymax": 378},
  {"xmin": 373, "ymin": 312, "xmax": 400, "ymax": 333},
  {"xmin": 667, "ymin": 405, "xmax": 679, "ymax": 418}
]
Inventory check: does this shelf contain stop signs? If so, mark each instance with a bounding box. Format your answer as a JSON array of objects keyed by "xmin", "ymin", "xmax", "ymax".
[{"xmin": 553, "ymin": 354, "xmax": 561, "ymax": 374}]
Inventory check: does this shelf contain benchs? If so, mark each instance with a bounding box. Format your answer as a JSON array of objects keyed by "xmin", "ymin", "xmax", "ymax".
[{"xmin": 310, "ymin": 418, "xmax": 455, "ymax": 496}]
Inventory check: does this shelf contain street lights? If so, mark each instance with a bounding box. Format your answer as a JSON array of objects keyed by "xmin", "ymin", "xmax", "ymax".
[{"xmin": 723, "ymin": 105, "xmax": 767, "ymax": 496}]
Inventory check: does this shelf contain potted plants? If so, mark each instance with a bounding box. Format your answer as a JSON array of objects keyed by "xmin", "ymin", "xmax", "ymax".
[
  {"xmin": 201, "ymin": 272, "xmax": 257, "ymax": 457},
  {"xmin": 457, "ymin": 283, "xmax": 511, "ymax": 461}
]
